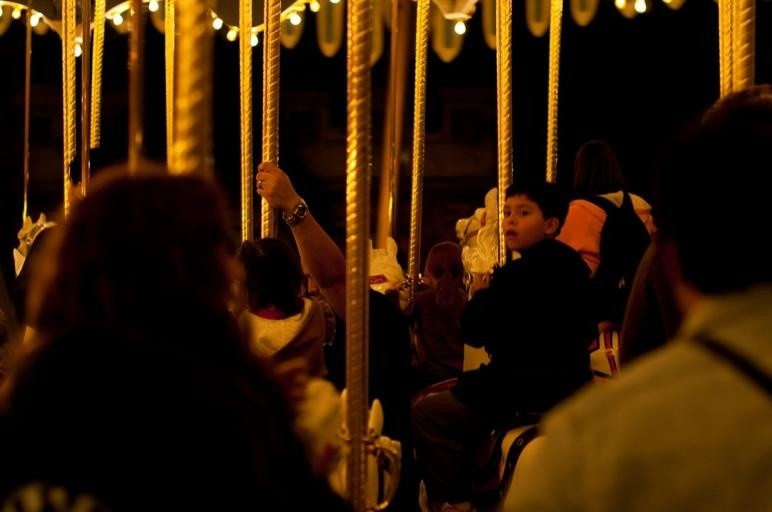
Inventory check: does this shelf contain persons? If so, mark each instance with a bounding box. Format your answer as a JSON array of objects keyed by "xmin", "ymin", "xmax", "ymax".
[
  {"xmin": 506, "ymin": 85, "xmax": 771, "ymax": 511},
  {"xmin": 0, "ymin": 138, "xmax": 688, "ymax": 512}
]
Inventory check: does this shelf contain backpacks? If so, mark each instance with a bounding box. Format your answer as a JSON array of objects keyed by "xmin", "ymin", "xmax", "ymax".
[{"xmin": 586, "ymin": 194, "xmax": 651, "ymax": 322}]
